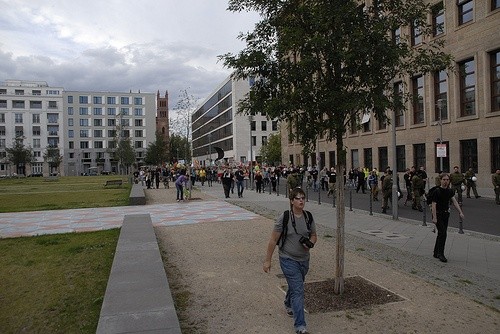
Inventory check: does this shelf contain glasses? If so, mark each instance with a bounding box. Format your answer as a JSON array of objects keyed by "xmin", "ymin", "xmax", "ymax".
[
  {"xmin": 295, "ymin": 197, "xmax": 305, "ymax": 200},
  {"xmin": 443, "ymin": 179, "xmax": 449, "ymax": 181}
]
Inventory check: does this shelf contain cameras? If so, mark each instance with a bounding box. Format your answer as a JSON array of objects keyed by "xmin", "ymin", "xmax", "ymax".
[{"xmin": 299, "ymin": 236, "xmax": 314, "ymax": 248}]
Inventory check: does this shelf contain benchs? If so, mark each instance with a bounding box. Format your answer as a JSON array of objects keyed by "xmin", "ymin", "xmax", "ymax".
[{"xmin": 102, "ymin": 179, "xmax": 123, "ymax": 189}]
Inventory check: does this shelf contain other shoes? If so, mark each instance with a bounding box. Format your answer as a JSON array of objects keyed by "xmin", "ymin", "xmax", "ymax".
[
  {"xmin": 306, "ymin": 186, "xmax": 427, "ymax": 214},
  {"xmin": 458, "ymin": 195, "xmax": 500, "ymax": 207},
  {"xmin": 296, "ymin": 328, "xmax": 310, "ymax": 334},
  {"xmin": 284, "ymin": 304, "xmax": 293, "ymax": 317},
  {"xmin": 433, "ymin": 252, "xmax": 447, "ymax": 263}
]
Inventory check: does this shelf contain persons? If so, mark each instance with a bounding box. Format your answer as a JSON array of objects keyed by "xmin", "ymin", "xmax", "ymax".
[
  {"xmin": 430, "ymin": 175, "xmax": 465, "ymax": 263},
  {"xmin": 263, "ymin": 188, "xmax": 318, "ymax": 334},
  {"xmin": 132, "ymin": 157, "xmax": 500, "ymax": 216},
  {"xmin": 39, "ymin": 170, "xmax": 113, "ymax": 177}
]
